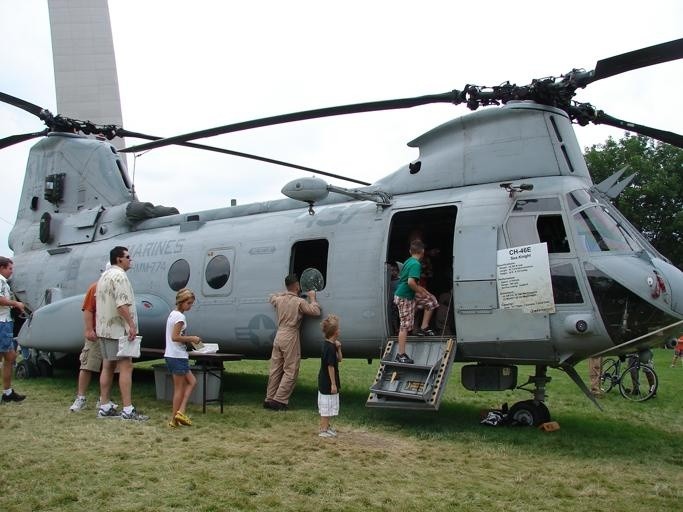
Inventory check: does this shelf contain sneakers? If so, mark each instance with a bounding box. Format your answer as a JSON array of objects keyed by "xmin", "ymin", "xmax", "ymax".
[
  {"xmin": 2, "ymin": 388, "xmax": 26, "ymax": 402},
  {"xmin": 263, "ymin": 400, "xmax": 288, "ymax": 410},
  {"xmin": 69, "ymin": 398, "xmax": 150, "ymax": 422},
  {"xmin": 394, "ymin": 352, "xmax": 414, "ymax": 364},
  {"xmin": 418, "ymin": 327, "xmax": 434, "ymax": 336},
  {"xmin": 318, "ymin": 430, "xmax": 336, "ymax": 438}
]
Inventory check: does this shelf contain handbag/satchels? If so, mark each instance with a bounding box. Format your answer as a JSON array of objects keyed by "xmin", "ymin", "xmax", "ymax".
[{"xmin": 11, "ymin": 306, "xmax": 32, "ymax": 337}]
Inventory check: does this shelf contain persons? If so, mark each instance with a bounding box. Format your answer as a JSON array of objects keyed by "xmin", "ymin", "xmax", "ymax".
[
  {"xmin": 11, "ymin": 308, "xmax": 29, "ymax": 368},
  {"xmin": 0, "ymin": 256, "xmax": 26, "ymax": 403},
  {"xmin": 671, "ymin": 335, "xmax": 683, "ymax": 367},
  {"xmin": 263, "ymin": 274, "xmax": 321, "ymax": 411},
  {"xmin": 588, "ymin": 357, "xmax": 604, "ymax": 395},
  {"xmin": 629, "ymin": 350, "xmax": 656, "ymax": 398},
  {"xmin": 95, "ymin": 246, "xmax": 151, "ymax": 422},
  {"xmin": 70, "ymin": 262, "xmax": 119, "ymax": 413},
  {"xmin": 163, "ymin": 288, "xmax": 203, "ymax": 428},
  {"xmin": 317, "ymin": 313, "xmax": 342, "ymax": 438},
  {"xmin": 393, "ymin": 240, "xmax": 441, "ymax": 364}
]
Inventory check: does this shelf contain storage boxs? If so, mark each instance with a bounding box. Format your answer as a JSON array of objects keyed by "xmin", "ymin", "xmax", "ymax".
[{"xmin": 152, "ymin": 362, "xmax": 225, "ymax": 406}]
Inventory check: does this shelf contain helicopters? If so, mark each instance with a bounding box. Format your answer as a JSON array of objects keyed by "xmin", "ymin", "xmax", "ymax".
[{"xmin": 0, "ymin": 38, "xmax": 682, "ymax": 429}]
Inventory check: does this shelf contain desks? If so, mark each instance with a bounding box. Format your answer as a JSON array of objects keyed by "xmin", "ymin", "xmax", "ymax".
[{"xmin": 141, "ymin": 347, "xmax": 246, "ymax": 414}]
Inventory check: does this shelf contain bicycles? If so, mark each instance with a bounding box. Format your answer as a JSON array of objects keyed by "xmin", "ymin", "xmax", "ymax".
[{"xmin": 599, "ymin": 350, "xmax": 658, "ymax": 402}]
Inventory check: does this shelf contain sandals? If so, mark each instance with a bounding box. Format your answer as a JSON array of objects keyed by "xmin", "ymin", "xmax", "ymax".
[{"xmin": 169, "ymin": 411, "xmax": 192, "ymax": 429}]
